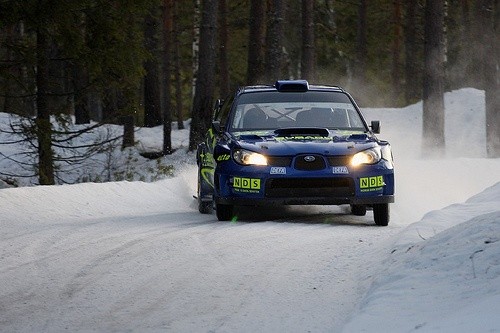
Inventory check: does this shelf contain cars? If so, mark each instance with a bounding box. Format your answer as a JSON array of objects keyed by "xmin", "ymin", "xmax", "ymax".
[{"xmin": 197, "ymin": 80, "xmax": 395, "ymax": 225}]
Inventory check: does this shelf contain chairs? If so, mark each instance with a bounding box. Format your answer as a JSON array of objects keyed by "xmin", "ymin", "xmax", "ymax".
[{"xmin": 243, "ymin": 107, "xmax": 356, "ymax": 131}]
